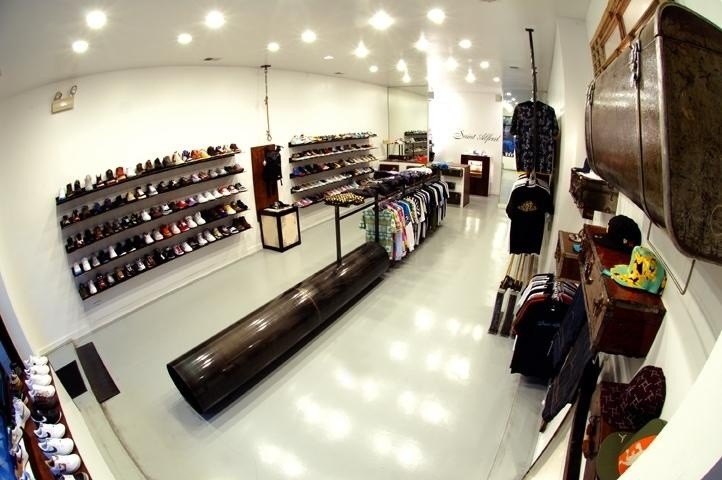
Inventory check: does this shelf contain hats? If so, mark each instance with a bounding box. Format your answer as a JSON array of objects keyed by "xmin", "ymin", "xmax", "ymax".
[
  {"xmin": 593, "ymin": 215, "xmax": 643, "ymax": 252},
  {"xmin": 596, "ymin": 418, "xmax": 668, "ymax": 480},
  {"xmin": 599, "ymin": 363, "xmax": 668, "ymax": 429},
  {"xmin": 610, "ymin": 247, "xmax": 668, "ymax": 296}
]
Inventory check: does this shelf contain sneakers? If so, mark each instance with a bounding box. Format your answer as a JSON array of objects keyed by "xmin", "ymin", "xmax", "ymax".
[
  {"xmin": 95, "ymin": 273, "xmax": 106, "ymax": 290},
  {"xmin": 67, "ymin": 183, "xmax": 74, "ymax": 199},
  {"xmin": 115, "ymin": 167, "xmax": 126, "ymax": 182},
  {"xmin": 99, "ymin": 250, "xmax": 108, "ymax": 264},
  {"xmin": 31, "ymin": 422, "xmax": 66, "ymax": 438},
  {"xmin": 121, "ymin": 144, "xmax": 254, "ymax": 293},
  {"xmin": 84, "ymin": 175, "xmax": 93, "ymax": 193},
  {"xmin": 72, "ymin": 208, "xmax": 81, "ymax": 223},
  {"xmin": 102, "ymin": 198, "xmax": 110, "ymax": 210},
  {"xmin": 78, "ymin": 282, "xmax": 89, "ymax": 300},
  {"xmin": 35, "ymin": 437, "xmax": 73, "ymax": 458},
  {"xmin": 74, "ymin": 179, "xmax": 82, "ymax": 195},
  {"xmin": 73, "ymin": 262, "xmax": 81, "ymax": 275},
  {"xmin": 87, "ymin": 279, "xmax": 97, "ymax": 295},
  {"xmin": 104, "ymin": 169, "xmax": 115, "ymax": 185},
  {"xmin": 290, "ymin": 128, "xmax": 394, "ymax": 210},
  {"xmin": 0, "ymin": 355, "xmax": 60, "ymax": 480},
  {"xmin": 62, "ymin": 214, "xmax": 70, "ymax": 226},
  {"xmin": 115, "ymin": 195, "xmax": 124, "ymax": 207},
  {"xmin": 57, "ymin": 188, "xmax": 66, "ymax": 201},
  {"xmin": 90, "ymin": 252, "xmax": 101, "ymax": 267},
  {"xmin": 95, "ymin": 174, "xmax": 104, "ymax": 188},
  {"xmin": 94, "ymin": 226, "xmax": 103, "ymax": 239},
  {"xmin": 107, "ymin": 246, "xmax": 119, "ymax": 260},
  {"xmin": 113, "ymin": 267, "xmax": 124, "ymax": 282},
  {"xmin": 84, "ymin": 229, "xmax": 93, "ymax": 244},
  {"xmin": 113, "ymin": 218, "xmax": 122, "ymax": 233},
  {"xmin": 94, "ymin": 201, "xmax": 100, "ymax": 216},
  {"xmin": 75, "ymin": 233, "xmax": 85, "ymax": 248},
  {"xmin": 115, "ymin": 242, "xmax": 126, "ymax": 257},
  {"xmin": 43, "ymin": 454, "xmax": 88, "ymax": 480},
  {"xmin": 81, "ymin": 205, "xmax": 91, "ymax": 219},
  {"xmin": 81, "ymin": 256, "xmax": 90, "ymax": 271},
  {"xmin": 103, "ymin": 222, "xmax": 113, "ymax": 236},
  {"xmin": 66, "ymin": 236, "xmax": 75, "ymax": 251}
]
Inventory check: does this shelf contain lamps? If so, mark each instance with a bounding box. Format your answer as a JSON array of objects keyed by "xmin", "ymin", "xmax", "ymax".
[{"xmin": 51, "ymin": 86, "xmax": 76, "ymax": 114}]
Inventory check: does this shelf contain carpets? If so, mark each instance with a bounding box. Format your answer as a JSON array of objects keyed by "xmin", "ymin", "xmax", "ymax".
[{"xmin": 76, "ymin": 341, "xmax": 121, "ymax": 402}]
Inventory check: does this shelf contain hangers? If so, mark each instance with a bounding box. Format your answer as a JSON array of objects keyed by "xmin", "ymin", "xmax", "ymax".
[
  {"xmin": 546, "ymin": 268, "xmax": 579, "ymax": 304},
  {"xmin": 522, "ymin": 173, "xmax": 543, "ymax": 190}
]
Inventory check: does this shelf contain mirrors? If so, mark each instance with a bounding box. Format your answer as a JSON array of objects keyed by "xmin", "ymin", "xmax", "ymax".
[{"xmin": 389, "ymin": 86, "xmax": 427, "ymax": 162}]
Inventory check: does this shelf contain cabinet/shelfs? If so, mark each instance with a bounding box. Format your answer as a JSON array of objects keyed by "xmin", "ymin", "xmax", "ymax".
[
  {"xmin": 57, "ymin": 149, "xmax": 252, "ymax": 301},
  {"xmin": 381, "ymin": 159, "xmax": 470, "ymax": 208},
  {"xmin": 288, "ymin": 134, "xmax": 374, "ymax": 209},
  {"xmin": 404, "ymin": 132, "xmax": 427, "ymax": 160}
]
